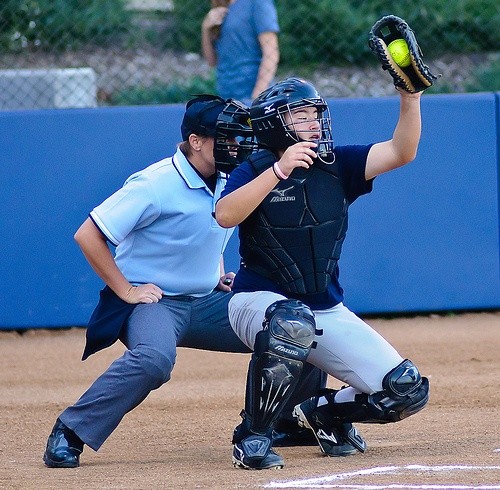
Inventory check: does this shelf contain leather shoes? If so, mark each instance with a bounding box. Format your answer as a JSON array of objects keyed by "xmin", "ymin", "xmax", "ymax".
[
  {"xmin": 43, "ymin": 430, "xmax": 83, "ymax": 468},
  {"xmin": 232, "ymin": 422, "xmax": 319, "ymax": 447}
]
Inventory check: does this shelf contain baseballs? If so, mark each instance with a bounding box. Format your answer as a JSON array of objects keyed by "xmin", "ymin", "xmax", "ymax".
[{"xmin": 386, "ymin": 39, "xmax": 410, "ymax": 67}]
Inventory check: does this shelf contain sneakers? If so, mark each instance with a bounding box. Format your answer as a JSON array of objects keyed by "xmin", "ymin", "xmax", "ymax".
[
  {"xmin": 292, "ymin": 395, "xmax": 357, "ymax": 457},
  {"xmin": 232, "ymin": 435, "xmax": 285, "ymax": 470}
]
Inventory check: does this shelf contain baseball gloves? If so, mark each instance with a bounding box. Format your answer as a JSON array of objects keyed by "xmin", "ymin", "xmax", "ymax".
[{"xmin": 369, "ymin": 15, "xmax": 433, "ymax": 95}]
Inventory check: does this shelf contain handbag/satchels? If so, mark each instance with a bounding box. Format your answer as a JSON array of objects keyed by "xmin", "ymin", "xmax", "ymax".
[{"xmin": 82, "ymin": 285, "xmax": 137, "ymax": 361}]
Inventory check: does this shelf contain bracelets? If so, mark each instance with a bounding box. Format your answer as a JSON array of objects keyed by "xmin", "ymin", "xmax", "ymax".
[{"xmin": 272, "ymin": 162, "xmax": 288, "ymax": 180}]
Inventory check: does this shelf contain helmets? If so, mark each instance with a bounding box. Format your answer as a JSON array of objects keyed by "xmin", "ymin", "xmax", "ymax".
[{"xmin": 249, "ymin": 77, "xmax": 335, "ymax": 164}]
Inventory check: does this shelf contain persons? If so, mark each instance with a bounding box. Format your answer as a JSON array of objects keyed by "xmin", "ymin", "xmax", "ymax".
[
  {"xmin": 44, "ymin": 94, "xmax": 326, "ymax": 468},
  {"xmin": 215, "ymin": 14, "xmax": 436, "ymax": 464},
  {"xmin": 201, "ymin": 0, "xmax": 281, "ymax": 101}
]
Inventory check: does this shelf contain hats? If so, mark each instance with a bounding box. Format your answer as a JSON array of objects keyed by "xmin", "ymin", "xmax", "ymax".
[{"xmin": 180, "ymin": 100, "xmax": 247, "ymax": 142}]
